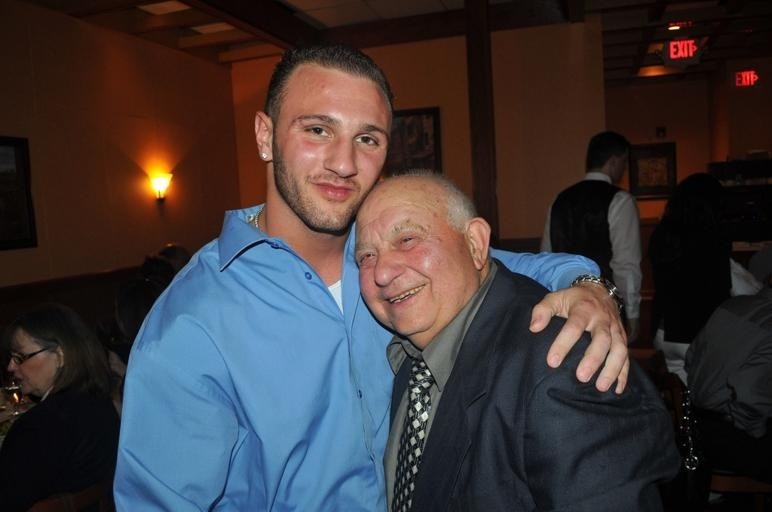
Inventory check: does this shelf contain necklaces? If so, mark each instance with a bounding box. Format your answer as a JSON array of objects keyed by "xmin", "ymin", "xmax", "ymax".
[{"xmin": 255, "ymin": 204, "xmax": 264, "ymax": 230}]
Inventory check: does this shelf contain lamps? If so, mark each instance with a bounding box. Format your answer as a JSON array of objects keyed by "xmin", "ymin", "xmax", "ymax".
[{"xmin": 149, "ymin": 173, "xmax": 173, "ymax": 205}]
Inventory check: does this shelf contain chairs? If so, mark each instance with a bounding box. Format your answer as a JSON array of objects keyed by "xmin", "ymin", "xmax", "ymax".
[
  {"xmin": 28, "ymin": 481, "xmax": 112, "ymax": 512},
  {"xmin": 671, "ymin": 378, "xmax": 771, "ymax": 510}
]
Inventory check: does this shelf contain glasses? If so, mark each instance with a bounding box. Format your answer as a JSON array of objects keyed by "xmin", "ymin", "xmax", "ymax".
[{"xmin": 11, "ymin": 347, "xmax": 49, "ymax": 365}]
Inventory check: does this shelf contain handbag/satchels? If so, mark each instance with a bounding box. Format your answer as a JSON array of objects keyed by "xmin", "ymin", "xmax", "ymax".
[{"xmin": 653, "ymin": 372, "xmax": 712, "ymax": 505}]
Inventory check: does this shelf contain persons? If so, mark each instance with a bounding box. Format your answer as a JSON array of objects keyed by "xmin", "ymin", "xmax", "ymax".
[
  {"xmin": 113, "ymin": 37, "xmax": 630, "ymax": 511},
  {"xmin": 354, "ymin": 163, "xmax": 703, "ymax": 511},
  {"xmin": 643, "ymin": 158, "xmax": 746, "ymax": 389},
  {"xmin": 1, "ymin": 302, "xmax": 120, "ymax": 512},
  {"xmin": 682, "ymin": 249, "xmax": 771, "ymax": 441},
  {"xmin": 107, "ymin": 245, "xmax": 190, "ymax": 408},
  {"xmin": 540, "ymin": 131, "xmax": 642, "ymax": 345}
]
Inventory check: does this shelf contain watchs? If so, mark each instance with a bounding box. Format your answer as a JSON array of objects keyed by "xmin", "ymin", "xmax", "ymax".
[{"xmin": 570, "ymin": 275, "xmax": 624, "ymax": 318}]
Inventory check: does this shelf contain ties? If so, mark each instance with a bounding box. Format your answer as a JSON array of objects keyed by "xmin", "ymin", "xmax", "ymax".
[{"xmin": 391, "ymin": 356, "xmax": 435, "ymax": 512}]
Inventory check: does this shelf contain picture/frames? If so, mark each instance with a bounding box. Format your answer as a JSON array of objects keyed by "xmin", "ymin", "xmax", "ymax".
[
  {"xmin": 628, "ymin": 143, "xmax": 678, "ymax": 200},
  {"xmin": 385, "ymin": 106, "xmax": 442, "ymax": 176},
  {"xmin": 0, "ymin": 134, "xmax": 38, "ymax": 251}
]
